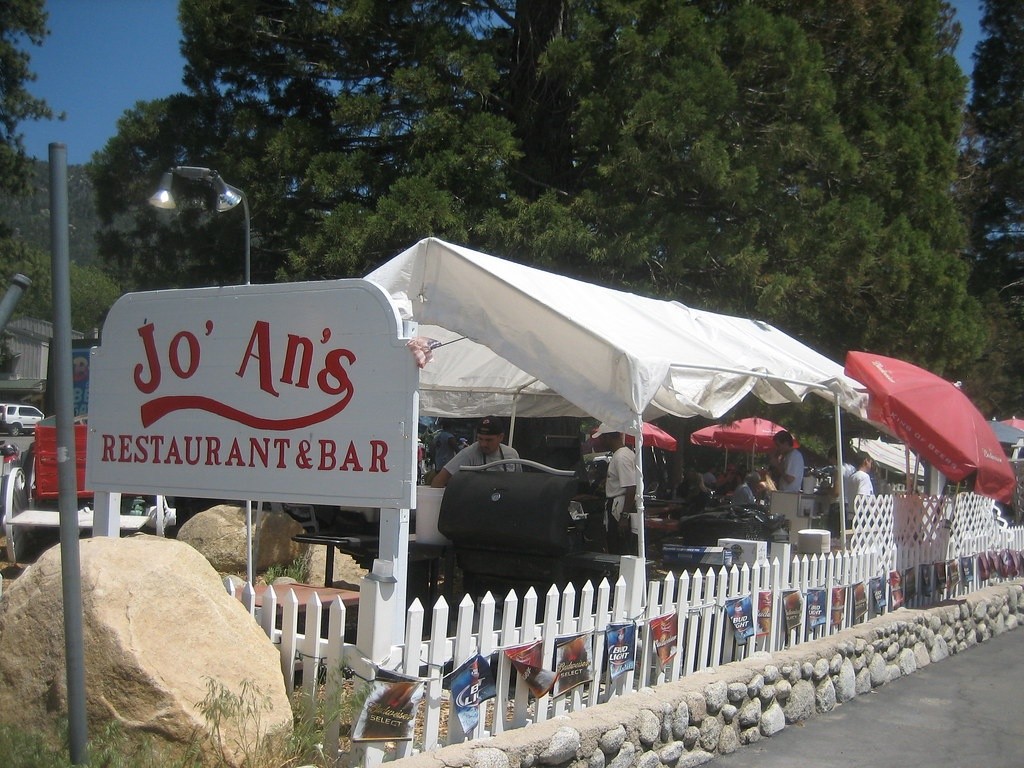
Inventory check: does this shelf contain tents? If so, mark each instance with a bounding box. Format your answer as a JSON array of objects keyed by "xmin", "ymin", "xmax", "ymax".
[{"xmin": 362, "ymin": 237, "xmax": 871, "ymax": 608}]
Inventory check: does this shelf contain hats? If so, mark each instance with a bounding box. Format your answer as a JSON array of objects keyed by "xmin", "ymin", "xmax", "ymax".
[
  {"xmin": 592, "ymin": 423, "xmax": 618, "ymax": 438},
  {"xmin": 477, "ymin": 415, "xmax": 503, "ymax": 435}
]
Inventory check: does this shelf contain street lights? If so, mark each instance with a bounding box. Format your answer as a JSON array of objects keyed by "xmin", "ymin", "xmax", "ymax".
[{"xmin": 148, "ymin": 164, "xmax": 251, "ymax": 287}]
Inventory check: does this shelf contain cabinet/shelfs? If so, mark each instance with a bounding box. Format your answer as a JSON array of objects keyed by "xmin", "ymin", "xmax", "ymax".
[{"xmin": 770, "ymin": 491, "xmax": 831, "ymax": 541}]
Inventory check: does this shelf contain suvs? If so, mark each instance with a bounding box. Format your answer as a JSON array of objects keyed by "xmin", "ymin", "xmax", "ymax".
[{"xmin": 0, "ymin": 404, "xmax": 45, "ymax": 437}]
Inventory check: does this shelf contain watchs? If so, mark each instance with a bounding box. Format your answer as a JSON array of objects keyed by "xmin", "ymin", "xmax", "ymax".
[{"xmin": 620, "ymin": 512, "xmax": 631, "ymax": 520}]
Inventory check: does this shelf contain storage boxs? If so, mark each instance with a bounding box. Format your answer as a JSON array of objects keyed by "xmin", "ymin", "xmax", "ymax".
[
  {"xmin": 717, "ymin": 537, "xmax": 768, "ymax": 566},
  {"xmin": 660, "ymin": 543, "xmax": 727, "ymax": 565}
]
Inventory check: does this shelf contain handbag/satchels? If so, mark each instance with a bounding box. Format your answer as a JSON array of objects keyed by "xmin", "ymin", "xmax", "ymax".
[{"xmin": 422, "ymin": 469, "xmax": 437, "ymax": 485}]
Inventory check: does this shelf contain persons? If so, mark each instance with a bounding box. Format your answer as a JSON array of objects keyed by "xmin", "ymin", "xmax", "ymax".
[
  {"xmin": 703, "ymin": 463, "xmax": 771, "ymax": 509},
  {"xmin": 429, "ymin": 414, "xmax": 522, "ymax": 489},
  {"xmin": 821, "ymin": 446, "xmax": 881, "ymax": 539},
  {"xmin": 685, "ymin": 464, "xmax": 710, "ymax": 514},
  {"xmin": 766, "ymin": 430, "xmax": 805, "ymax": 493},
  {"xmin": 429, "ymin": 418, "xmax": 460, "ymax": 473},
  {"xmin": 591, "ymin": 422, "xmax": 638, "ymax": 556},
  {"xmin": 0, "ymin": 439, "xmax": 20, "ymax": 462}
]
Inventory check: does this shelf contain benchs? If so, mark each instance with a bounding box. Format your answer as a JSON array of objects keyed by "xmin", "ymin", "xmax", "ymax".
[{"xmin": 6, "ymin": 510, "xmax": 150, "ymax": 532}]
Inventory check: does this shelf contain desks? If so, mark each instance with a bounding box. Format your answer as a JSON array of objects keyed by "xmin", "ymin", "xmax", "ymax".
[{"xmin": 233, "ymin": 582, "xmax": 360, "ymax": 638}]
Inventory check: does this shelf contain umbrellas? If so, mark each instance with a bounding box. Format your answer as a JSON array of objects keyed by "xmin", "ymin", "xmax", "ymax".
[
  {"xmin": 713, "ymin": 416, "xmax": 801, "ymax": 472},
  {"xmin": 849, "ymin": 436, "xmax": 925, "ymax": 479},
  {"xmin": 841, "ymin": 349, "xmax": 1015, "ymax": 506},
  {"xmin": 689, "ymin": 417, "xmax": 745, "ymax": 472},
  {"xmin": 626, "ymin": 421, "xmax": 677, "ymax": 454},
  {"xmin": 1002, "ymin": 416, "xmax": 1024, "ymax": 431},
  {"xmin": 984, "ymin": 416, "xmax": 1024, "ymax": 444}
]
